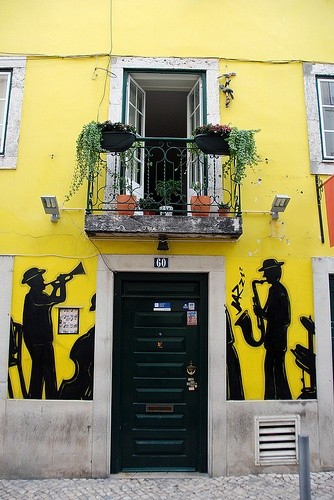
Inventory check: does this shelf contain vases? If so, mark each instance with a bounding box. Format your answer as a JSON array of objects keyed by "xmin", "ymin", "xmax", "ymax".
[
  {"xmin": 193, "ymin": 133, "xmax": 230, "ymax": 157},
  {"xmin": 100, "ymin": 132, "xmax": 136, "ymax": 153},
  {"xmin": 117, "ymin": 195, "xmax": 137, "ymax": 216}
]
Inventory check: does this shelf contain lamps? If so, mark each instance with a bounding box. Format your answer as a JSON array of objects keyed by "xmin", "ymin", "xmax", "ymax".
[
  {"xmin": 41, "ymin": 196, "xmax": 60, "ymax": 223},
  {"xmin": 270, "ymin": 194, "xmax": 291, "ymax": 220}
]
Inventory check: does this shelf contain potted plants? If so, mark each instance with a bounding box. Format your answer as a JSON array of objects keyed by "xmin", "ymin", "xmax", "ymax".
[
  {"xmin": 218, "ymin": 203, "xmax": 230, "ymax": 217},
  {"xmin": 140, "ymin": 197, "xmax": 155, "ymax": 215},
  {"xmin": 156, "ymin": 179, "xmax": 181, "ymax": 217},
  {"xmin": 190, "ymin": 177, "xmax": 212, "ymax": 217}
]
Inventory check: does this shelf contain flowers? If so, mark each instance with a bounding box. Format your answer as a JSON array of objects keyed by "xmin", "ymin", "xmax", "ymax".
[
  {"xmin": 188, "ymin": 123, "xmax": 268, "ymax": 186},
  {"xmin": 63, "ymin": 119, "xmax": 143, "ymax": 213}
]
